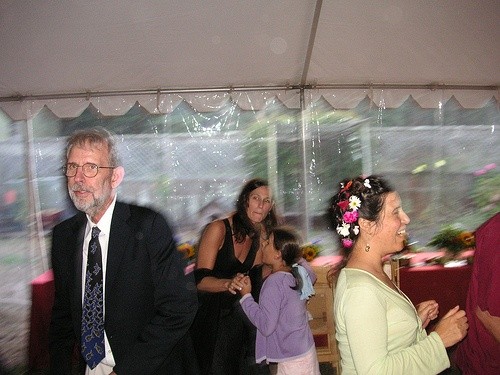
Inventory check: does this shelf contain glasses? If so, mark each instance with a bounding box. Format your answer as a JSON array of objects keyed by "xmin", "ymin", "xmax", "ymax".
[{"xmin": 62, "ymin": 163, "xmax": 116, "ymax": 177}]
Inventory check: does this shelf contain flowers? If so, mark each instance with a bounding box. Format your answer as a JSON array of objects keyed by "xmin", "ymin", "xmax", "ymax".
[
  {"xmin": 336, "ymin": 177, "xmax": 372, "ymax": 247},
  {"xmin": 460, "ymin": 232, "xmax": 474, "ymax": 244},
  {"xmin": 177, "ymin": 244, "xmax": 194, "ymax": 258},
  {"xmin": 300, "ymin": 246, "xmax": 317, "ymax": 261}
]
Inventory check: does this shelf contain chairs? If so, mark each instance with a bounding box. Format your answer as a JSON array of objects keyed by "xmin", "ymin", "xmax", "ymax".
[{"xmin": 306, "ymin": 283, "xmax": 340, "ymax": 375}]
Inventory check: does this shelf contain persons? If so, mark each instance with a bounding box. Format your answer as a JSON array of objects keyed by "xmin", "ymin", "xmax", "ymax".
[
  {"xmin": 48, "ymin": 125, "xmax": 199, "ymax": 375},
  {"xmin": 239, "ymin": 229, "xmax": 321, "ymax": 375},
  {"xmin": 327, "ymin": 175, "xmax": 469, "ymax": 375},
  {"xmin": 194, "ymin": 179, "xmax": 285, "ymax": 375}
]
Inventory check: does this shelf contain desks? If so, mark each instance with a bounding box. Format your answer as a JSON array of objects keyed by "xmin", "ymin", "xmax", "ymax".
[{"xmin": 399, "ymin": 251, "xmax": 473, "ymax": 321}]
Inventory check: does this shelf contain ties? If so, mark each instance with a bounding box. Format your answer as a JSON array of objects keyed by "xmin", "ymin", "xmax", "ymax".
[{"xmin": 82, "ymin": 226, "xmax": 105, "ymax": 370}]
